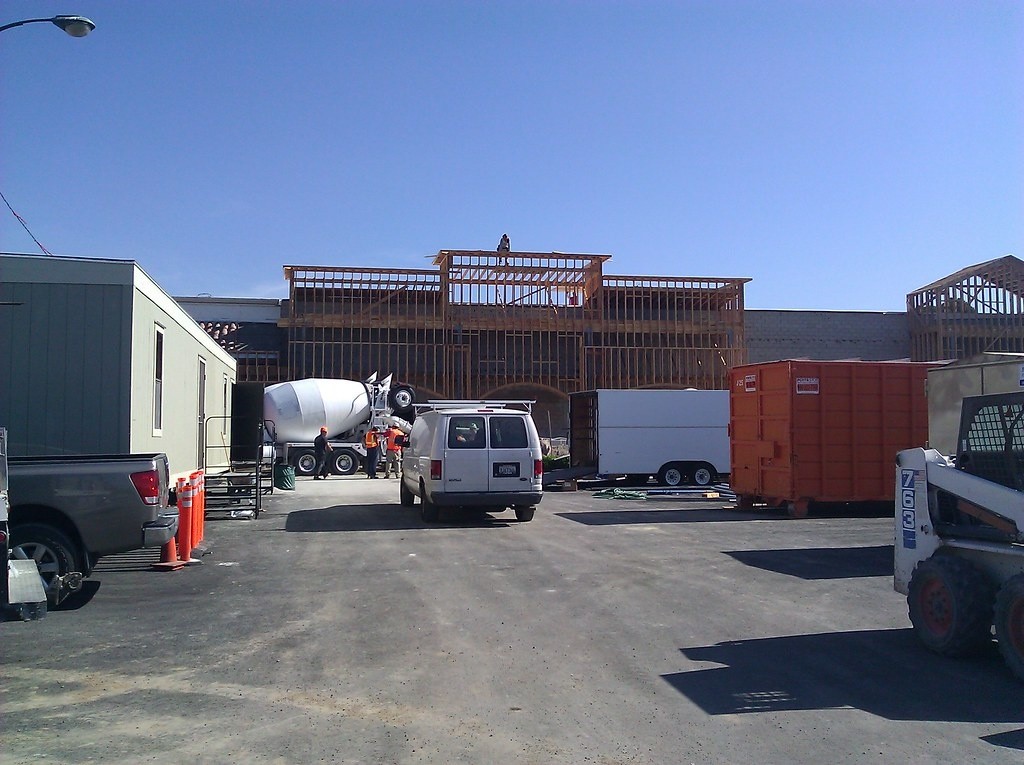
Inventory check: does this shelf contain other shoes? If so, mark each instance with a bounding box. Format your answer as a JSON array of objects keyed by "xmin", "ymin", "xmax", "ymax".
[
  {"xmin": 384, "ymin": 476, "xmax": 389, "ymax": 479},
  {"xmin": 314, "ymin": 476, "xmax": 321, "ymax": 480},
  {"xmin": 371, "ymin": 476, "xmax": 378, "ymax": 479}
]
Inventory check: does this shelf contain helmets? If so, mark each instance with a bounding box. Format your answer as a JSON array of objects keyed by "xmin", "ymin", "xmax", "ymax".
[
  {"xmin": 393, "ymin": 422, "xmax": 399, "ymax": 427},
  {"xmin": 321, "ymin": 427, "xmax": 328, "ymax": 433}
]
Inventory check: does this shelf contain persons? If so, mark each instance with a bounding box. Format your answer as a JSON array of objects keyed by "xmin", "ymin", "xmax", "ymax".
[
  {"xmin": 314, "ymin": 427, "xmax": 333, "ymax": 480},
  {"xmin": 362, "ymin": 426, "xmax": 381, "ymax": 479},
  {"xmin": 567, "ymin": 291, "xmax": 578, "ymax": 305},
  {"xmin": 381, "ymin": 423, "xmax": 405, "ymax": 479},
  {"xmin": 497, "ymin": 235, "xmax": 511, "ymax": 265},
  {"xmin": 458, "ymin": 423, "xmax": 477, "ymax": 443}
]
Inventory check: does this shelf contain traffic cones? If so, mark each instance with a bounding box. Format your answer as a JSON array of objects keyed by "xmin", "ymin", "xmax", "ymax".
[{"xmin": 151, "ymin": 537, "xmax": 185, "ymax": 570}]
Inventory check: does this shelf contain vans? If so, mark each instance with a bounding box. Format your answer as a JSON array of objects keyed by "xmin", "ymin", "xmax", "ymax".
[{"xmin": 394, "ymin": 399, "xmax": 542, "ymax": 522}]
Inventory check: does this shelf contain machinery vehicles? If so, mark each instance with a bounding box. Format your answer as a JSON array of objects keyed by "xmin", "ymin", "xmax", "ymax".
[
  {"xmin": 895, "ymin": 391, "xmax": 1024, "ymax": 678},
  {"xmin": 264, "ymin": 372, "xmax": 393, "ymax": 475}
]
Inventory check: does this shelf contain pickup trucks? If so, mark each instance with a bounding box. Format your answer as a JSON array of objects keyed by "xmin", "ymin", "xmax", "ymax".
[{"xmin": 0, "ymin": 427, "xmax": 180, "ymax": 611}]
